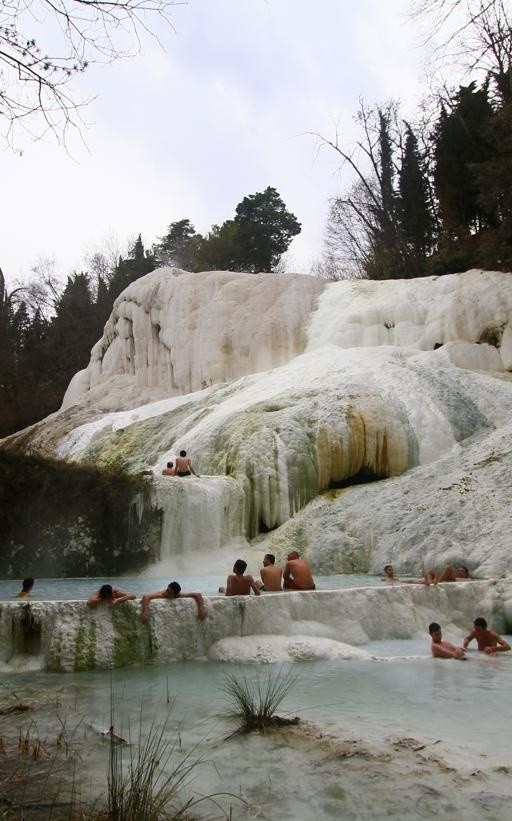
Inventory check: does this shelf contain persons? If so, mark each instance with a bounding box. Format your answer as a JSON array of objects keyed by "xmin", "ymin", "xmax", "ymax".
[
  {"xmin": 381, "ymin": 564, "xmax": 437, "ymax": 586},
  {"xmin": 173, "ymin": 451, "xmax": 201, "ymax": 478},
  {"xmin": 219, "ymin": 560, "xmax": 261, "ymax": 596},
  {"xmin": 162, "ymin": 463, "xmax": 176, "ymax": 477},
  {"xmin": 16, "ymin": 577, "xmax": 34, "ymax": 599},
  {"xmin": 283, "ymin": 552, "xmax": 315, "ymax": 591},
  {"xmin": 255, "ymin": 554, "xmax": 282, "ymax": 592},
  {"xmin": 428, "ymin": 566, "xmax": 469, "ymax": 585},
  {"xmin": 142, "ymin": 582, "xmax": 207, "ymax": 625},
  {"xmin": 87, "ymin": 585, "xmax": 137, "ymax": 609},
  {"xmin": 463, "ymin": 618, "xmax": 511, "ymax": 654},
  {"xmin": 428, "ymin": 623, "xmax": 465, "ymax": 658}
]
